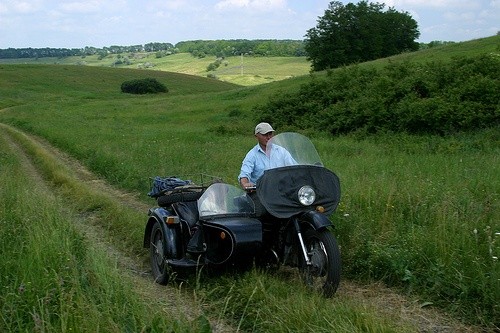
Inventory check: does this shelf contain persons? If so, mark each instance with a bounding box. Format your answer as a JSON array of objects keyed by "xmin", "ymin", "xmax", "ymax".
[{"xmin": 238, "ymin": 122, "xmax": 300, "ymax": 217}]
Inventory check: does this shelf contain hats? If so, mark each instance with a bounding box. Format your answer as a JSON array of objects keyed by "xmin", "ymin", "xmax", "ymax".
[{"xmin": 255, "ymin": 122, "xmax": 275, "ymax": 135}]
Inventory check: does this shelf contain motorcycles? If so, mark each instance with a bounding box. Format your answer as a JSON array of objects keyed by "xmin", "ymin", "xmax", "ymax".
[{"xmin": 142, "ymin": 132, "xmax": 342, "ymax": 298}]
